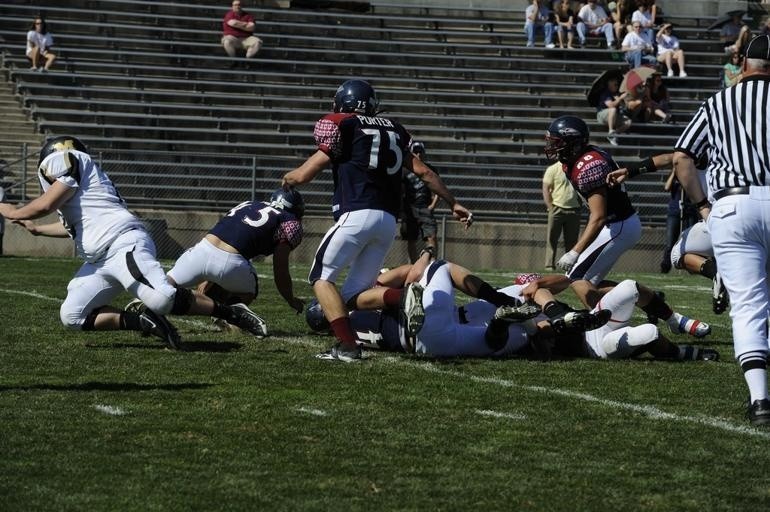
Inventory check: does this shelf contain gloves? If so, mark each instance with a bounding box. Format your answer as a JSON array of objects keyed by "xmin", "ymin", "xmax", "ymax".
[{"xmin": 556, "ymin": 250, "xmax": 580, "ymax": 275}]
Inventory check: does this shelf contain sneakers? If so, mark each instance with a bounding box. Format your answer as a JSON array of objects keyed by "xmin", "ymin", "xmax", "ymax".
[
  {"xmin": 665, "ymin": 115, "xmax": 674, "ymax": 123},
  {"xmin": 545, "ymin": 43, "xmax": 556, "ymax": 48},
  {"xmin": 645, "ymin": 292, "xmax": 664, "ymax": 323},
  {"xmin": 745, "ymin": 399, "xmax": 769, "ymax": 425},
  {"xmin": 527, "ymin": 41, "xmax": 534, "ymax": 47},
  {"xmin": 608, "ymin": 129, "xmax": 616, "ymax": 134},
  {"xmin": 668, "ymin": 70, "xmax": 673, "ymax": 77},
  {"xmin": 140, "ymin": 308, "xmax": 182, "ymax": 351},
  {"xmin": 607, "ymin": 133, "xmax": 619, "ymax": 146},
  {"xmin": 314, "ymin": 342, "xmax": 360, "ymax": 363},
  {"xmin": 669, "ymin": 313, "xmax": 711, "ymax": 337},
  {"xmin": 227, "ymin": 304, "xmax": 267, "ymax": 338},
  {"xmin": 124, "ymin": 300, "xmax": 147, "ymax": 314},
  {"xmin": 679, "ymin": 346, "xmax": 719, "ymax": 362},
  {"xmin": 495, "ymin": 298, "xmax": 611, "ymax": 336},
  {"xmin": 679, "ymin": 71, "xmax": 688, "ymax": 77},
  {"xmin": 402, "ymin": 282, "xmax": 425, "ymax": 336},
  {"xmin": 712, "ymin": 264, "xmax": 729, "ymax": 315}
]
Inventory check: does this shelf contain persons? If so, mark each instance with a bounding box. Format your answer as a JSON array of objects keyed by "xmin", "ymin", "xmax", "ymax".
[
  {"xmin": 672, "ymin": 34, "xmax": 769, "ymax": 429},
  {"xmin": 0, "ymin": 137, "xmax": 268, "ymax": 351},
  {"xmin": 124, "ymin": 189, "xmax": 307, "ymax": 333},
  {"xmin": 25, "ymin": 12, "xmax": 56, "ymax": 73},
  {"xmin": 221, "ymin": 0, "xmax": 265, "ymax": 58}
]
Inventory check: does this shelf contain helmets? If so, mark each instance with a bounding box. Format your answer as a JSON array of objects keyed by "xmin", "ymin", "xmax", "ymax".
[
  {"xmin": 306, "ymin": 297, "xmax": 330, "ymax": 331},
  {"xmin": 333, "ymin": 79, "xmax": 379, "ymax": 115},
  {"xmin": 544, "ymin": 117, "xmax": 589, "ymax": 162},
  {"xmin": 271, "ymin": 187, "xmax": 304, "ymax": 218},
  {"xmin": 39, "ymin": 136, "xmax": 86, "ymax": 165}
]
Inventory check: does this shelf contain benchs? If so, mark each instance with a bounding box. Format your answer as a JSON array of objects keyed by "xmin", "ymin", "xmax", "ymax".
[{"xmin": 0, "ymin": 0, "xmax": 770, "ymax": 220}]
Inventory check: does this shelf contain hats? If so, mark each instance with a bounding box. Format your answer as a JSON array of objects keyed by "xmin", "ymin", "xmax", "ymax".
[
  {"xmin": 745, "ymin": 34, "xmax": 770, "ymax": 60},
  {"xmin": 412, "ymin": 142, "xmax": 426, "ymax": 154}
]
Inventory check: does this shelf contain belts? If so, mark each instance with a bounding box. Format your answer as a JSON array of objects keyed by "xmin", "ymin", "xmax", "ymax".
[{"xmin": 713, "ymin": 186, "xmax": 749, "ymax": 200}]
